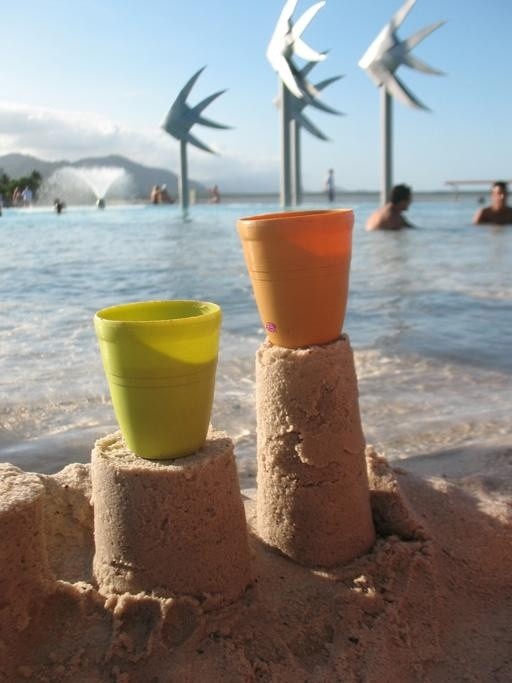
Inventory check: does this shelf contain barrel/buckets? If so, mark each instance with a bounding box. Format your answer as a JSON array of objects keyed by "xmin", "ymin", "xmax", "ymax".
[
  {"xmin": 236, "ymin": 209, "xmax": 356, "ymax": 350},
  {"xmin": 94, "ymin": 303, "xmax": 223, "ymax": 459}
]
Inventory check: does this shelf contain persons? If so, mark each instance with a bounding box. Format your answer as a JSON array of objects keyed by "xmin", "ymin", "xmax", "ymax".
[
  {"xmin": 471, "ymin": 180, "xmax": 512, "ymax": 225},
  {"xmin": 209, "ymin": 185, "xmax": 221, "ymax": 203},
  {"xmin": 150, "ymin": 184, "xmax": 161, "ymax": 205},
  {"xmin": 158, "ymin": 183, "xmax": 175, "ymax": 203},
  {"xmin": 363, "ymin": 183, "xmax": 415, "ymax": 231},
  {"xmin": 21, "ymin": 184, "xmax": 34, "ymax": 209},
  {"xmin": 12, "ymin": 184, "xmax": 21, "ymax": 208},
  {"xmin": 326, "ymin": 168, "xmax": 335, "ymax": 202}
]
